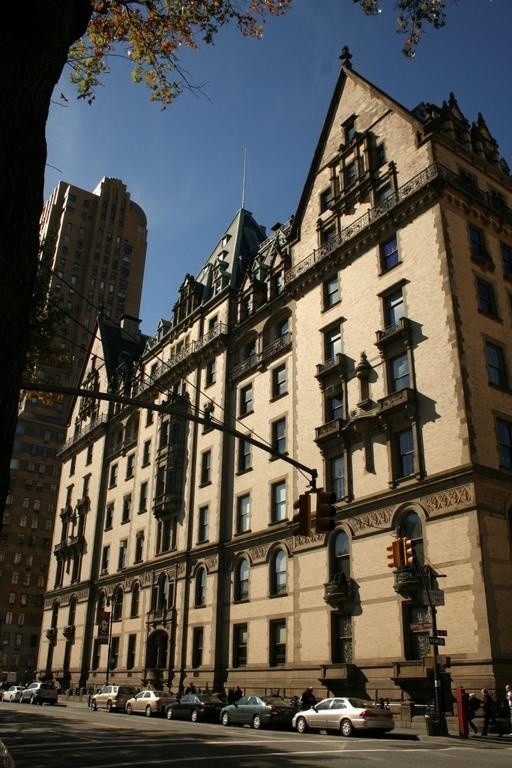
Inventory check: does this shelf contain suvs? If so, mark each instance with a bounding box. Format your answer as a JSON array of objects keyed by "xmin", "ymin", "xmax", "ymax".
[
  {"xmin": 18, "ymin": 681, "xmax": 58, "ymax": 705},
  {"xmin": 89, "ymin": 684, "xmax": 137, "ymax": 712}
]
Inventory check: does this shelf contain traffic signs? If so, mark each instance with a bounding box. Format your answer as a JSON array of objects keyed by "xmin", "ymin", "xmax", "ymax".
[
  {"xmin": 429, "ymin": 636, "xmax": 445, "ymax": 645},
  {"xmin": 434, "ymin": 629, "xmax": 447, "ymax": 636},
  {"xmin": 422, "ymin": 589, "xmax": 444, "ymax": 605},
  {"xmin": 410, "ymin": 623, "xmax": 432, "ymax": 631}
]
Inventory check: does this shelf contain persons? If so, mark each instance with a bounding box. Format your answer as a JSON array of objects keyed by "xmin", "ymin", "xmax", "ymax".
[
  {"xmin": 462, "ymin": 690, "xmax": 480, "ymax": 735},
  {"xmin": 299, "ymin": 686, "xmax": 318, "ymax": 711},
  {"xmin": 480, "ymin": 687, "xmax": 505, "ymax": 736},
  {"xmin": 186, "ymin": 683, "xmax": 244, "ymax": 705},
  {"xmin": 506, "ymin": 683, "xmax": 512, "ymax": 723}
]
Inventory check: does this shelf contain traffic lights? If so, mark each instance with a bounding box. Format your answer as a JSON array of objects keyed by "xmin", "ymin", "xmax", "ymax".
[
  {"xmin": 387, "ymin": 537, "xmax": 404, "ymax": 567},
  {"xmin": 316, "ymin": 490, "xmax": 337, "ymax": 530},
  {"xmin": 403, "ymin": 536, "xmax": 416, "ymax": 565},
  {"xmin": 293, "ymin": 494, "xmax": 312, "ymax": 537}
]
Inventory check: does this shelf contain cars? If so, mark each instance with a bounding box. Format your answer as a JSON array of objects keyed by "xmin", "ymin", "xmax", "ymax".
[
  {"xmin": 220, "ymin": 694, "xmax": 295, "ymax": 729},
  {"xmin": 124, "ymin": 690, "xmax": 177, "ymax": 716},
  {"xmin": 1, "ymin": 681, "xmax": 16, "ymax": 690},
  {"xmin": 291, "ymin": 696, "xmax": 395, "ymax": 736},
  {"xmin": 1, "ymin": 685, "xmax": 25, "ymax": 702},
  {"xmin": 0, "ymin": 737, "xmax": 16, "ymax": 767},
  {"xmin": 164, "ymin": 693, "xmax": 225, "ymax": 722}
]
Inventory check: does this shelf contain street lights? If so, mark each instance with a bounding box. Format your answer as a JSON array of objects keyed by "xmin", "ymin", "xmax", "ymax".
[{"xmin": 106, "ymin": 595, "xmax": 118, "ymax": 684}]
